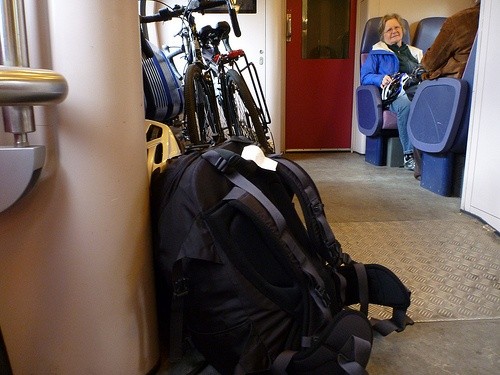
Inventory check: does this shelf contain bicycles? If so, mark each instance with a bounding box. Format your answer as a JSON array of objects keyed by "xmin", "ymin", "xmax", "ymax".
[{"xmin": 139, "ymin": 0, "xmax": 277, "ymax": 156}]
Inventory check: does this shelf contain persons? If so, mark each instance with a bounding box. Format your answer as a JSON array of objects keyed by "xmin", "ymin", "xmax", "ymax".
[
  {"xmin": 420, "ymin": 0, "xmax": 482, "ymax": 80},
  {"xmin": 360, "ymin": 13, "xmax": 426, "ymax": 172}
]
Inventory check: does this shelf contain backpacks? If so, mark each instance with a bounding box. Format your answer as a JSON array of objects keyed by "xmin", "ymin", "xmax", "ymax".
[{"xmin": 150, "ymin": 137, "xmax": 414, "ymax": 375}]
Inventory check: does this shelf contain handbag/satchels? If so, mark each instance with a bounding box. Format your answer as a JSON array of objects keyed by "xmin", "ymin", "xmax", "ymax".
[
  {"xmin": 381, "ymin": 66, "xmax": 426, "ymax": 106},
  {"xmin": 141, "ymin": 50, "xmax": 184, "ymax": 122}
]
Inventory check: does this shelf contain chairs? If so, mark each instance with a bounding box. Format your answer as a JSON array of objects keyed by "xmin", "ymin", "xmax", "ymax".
[{"xmin": 356, "ymin": 16, "xmax": 478, "ymax": 197}]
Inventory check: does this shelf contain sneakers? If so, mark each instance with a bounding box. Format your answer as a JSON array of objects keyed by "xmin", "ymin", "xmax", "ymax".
[{"xmin": 403, "ymin": 154, "xmax": 415, "ymax": 171}]
